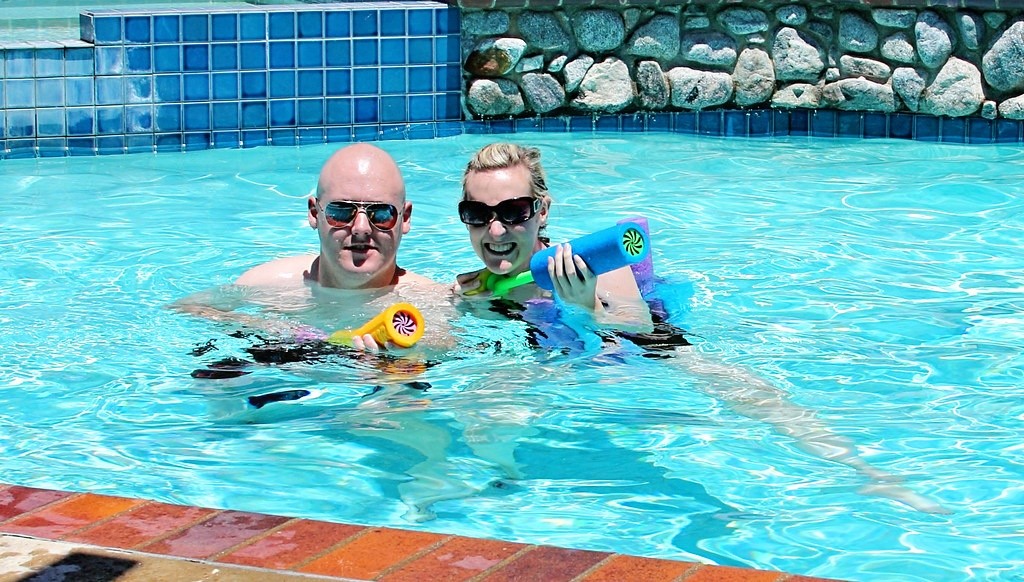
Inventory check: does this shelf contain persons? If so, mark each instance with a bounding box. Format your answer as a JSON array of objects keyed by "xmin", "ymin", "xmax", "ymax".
[
  {"xmin": 236, "ymin": 142, "xmax": 439, "ymax": 287},
  {"xmin": 446, "ymin": 142, "xmax": 643, "ymax": 313}
]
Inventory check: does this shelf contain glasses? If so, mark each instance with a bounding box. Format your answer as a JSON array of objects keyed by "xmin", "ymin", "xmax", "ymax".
[
  {"xmin": 458, "ymin": 196, "xmax": 543, "ymax": 225},
  {"xmin": 314, "ymin": 200, "xmax": 405, "ymax": 232}
]
